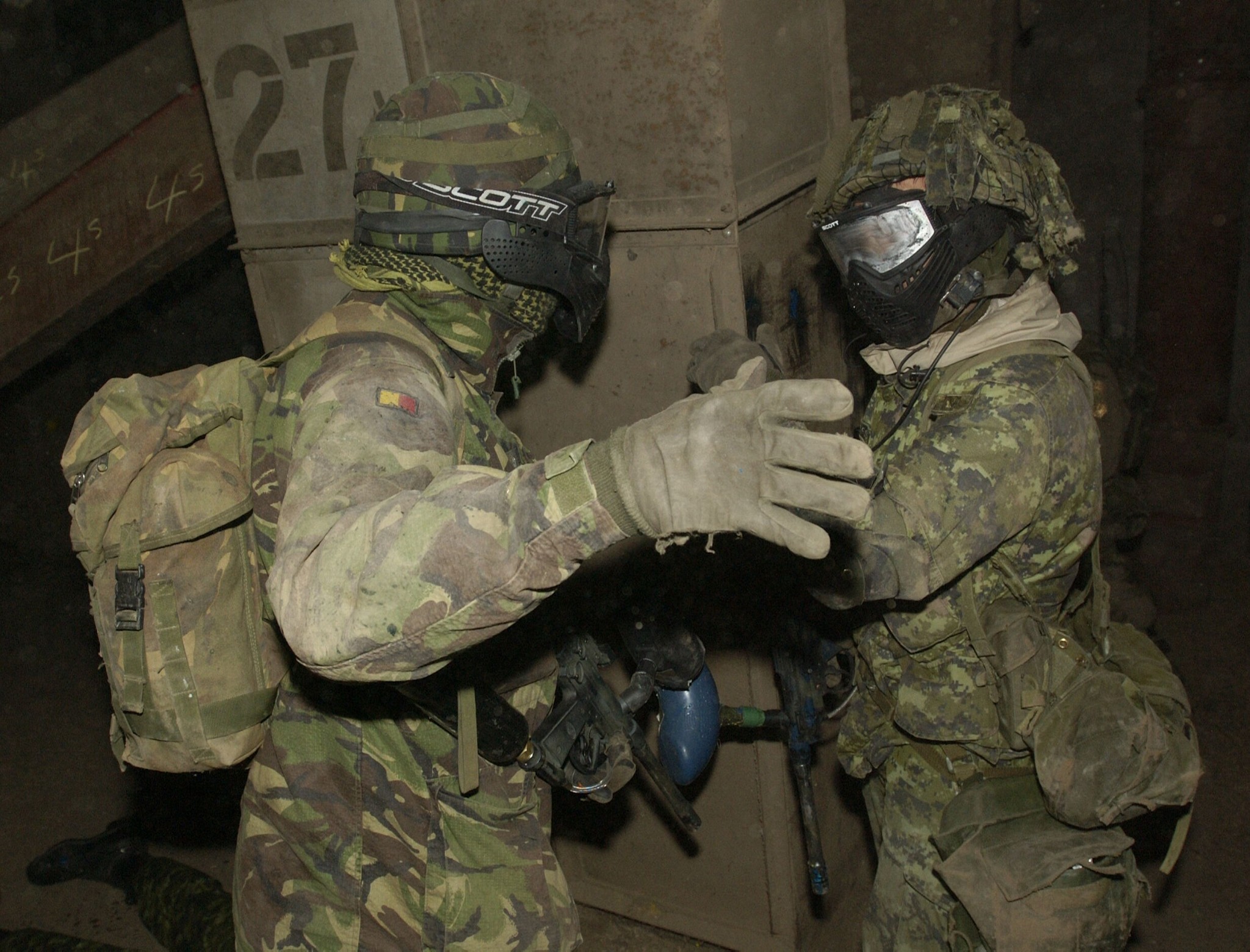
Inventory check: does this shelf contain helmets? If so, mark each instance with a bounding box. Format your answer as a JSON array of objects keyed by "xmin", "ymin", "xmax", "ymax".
[
  {"xmin": 808, "ymin": 87, "xmax": 1085, "ymax": 252},
  {"xmin": 353, "ymin": 72, "xmax": 581, "ymax": 256}
]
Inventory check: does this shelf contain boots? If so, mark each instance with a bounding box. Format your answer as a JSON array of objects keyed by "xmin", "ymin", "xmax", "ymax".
[{"xmin": 27, "ymin": 816, "xmax": 153, "ymax": 894}]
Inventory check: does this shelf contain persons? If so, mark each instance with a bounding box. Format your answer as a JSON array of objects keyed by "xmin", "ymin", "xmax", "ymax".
[
  {"xmin": 684, "ymin": 81, "xmax": 1204, "ymax": 952},
  {"xmin": 0, "ymin": 67, "xmax": 875, "ymax": 952}
]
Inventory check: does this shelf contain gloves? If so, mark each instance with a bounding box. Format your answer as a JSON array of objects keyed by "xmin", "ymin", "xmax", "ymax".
[{"xmin": 622, "ymin": 356, "xmax": 874, "ymax": 560}]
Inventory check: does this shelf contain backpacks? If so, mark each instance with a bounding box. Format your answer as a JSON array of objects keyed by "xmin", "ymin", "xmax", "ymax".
[{"xmin": 59, "ymin": 303, "xmax": 465, "ymax": 772}]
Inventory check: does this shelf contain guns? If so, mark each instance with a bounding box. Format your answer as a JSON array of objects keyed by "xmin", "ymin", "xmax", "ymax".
[
  {"xmin": 522, "ymin": 601, "xmax": 707, "ymax": 832},
  {"xmin": 768, "ymin": 527, "xmax": 844, "ymax": 909}
]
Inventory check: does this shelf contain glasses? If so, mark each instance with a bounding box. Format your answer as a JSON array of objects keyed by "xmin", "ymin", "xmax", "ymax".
[
  {"xmin": 564, "ymin": 182, "xmax": 614, "ymax": 268},
  {"xmin": 814, "ymin": 191, "xmax": 943, "ymax": 284}
]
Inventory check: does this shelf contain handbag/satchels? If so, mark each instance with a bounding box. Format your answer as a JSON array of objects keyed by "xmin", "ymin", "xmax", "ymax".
[
  {"xmin": 990, "ymin": 599, "xmax": 1201, "ymax": 828},
  {"xmin": 935, "ymin": 774, "xmax": 1150, "ymax": 952}
]
